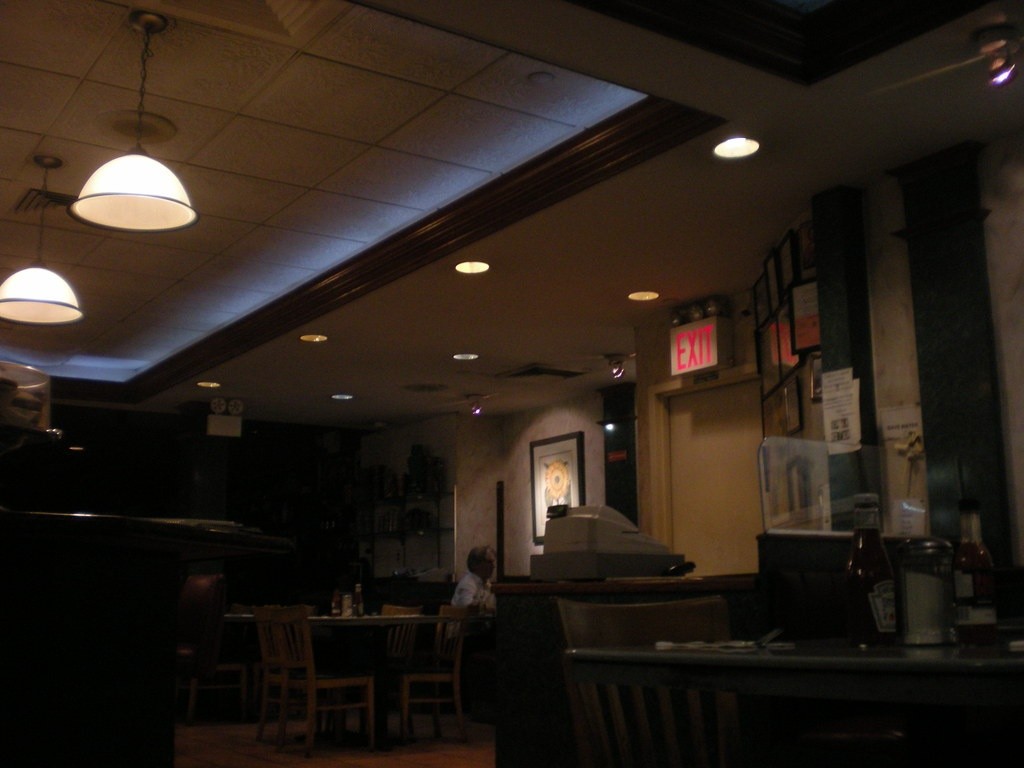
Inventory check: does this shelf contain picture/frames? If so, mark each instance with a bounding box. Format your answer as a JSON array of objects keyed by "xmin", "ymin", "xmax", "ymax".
[
  {"xmin": 528, "ymin": 431, "xmax": 585, "ymax": 545},
  {"xmin": 750, "ymin": 228, "xmax": 823, "ymax": 436}
]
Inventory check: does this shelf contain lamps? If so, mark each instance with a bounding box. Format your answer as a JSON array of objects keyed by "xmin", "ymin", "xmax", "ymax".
[
  {"xmin": 669, "ymin": 293, "xmax": 730, "ymax": 328},
  {"xmin": 0, "ymin": 154, "xmax": 83, "ymax": 325},
  {"xmin": 207, "ymin": 397, "xmax": 243, "ymax": 437},
  {"xmin": 67, "ymin": 10, "xmax": 199, "ymax": 233}
]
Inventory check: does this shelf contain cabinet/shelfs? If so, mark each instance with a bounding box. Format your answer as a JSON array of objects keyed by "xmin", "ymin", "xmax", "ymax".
[{"xmin": 350, "ymin": 489, "xmax": 454, "ymax": 541}]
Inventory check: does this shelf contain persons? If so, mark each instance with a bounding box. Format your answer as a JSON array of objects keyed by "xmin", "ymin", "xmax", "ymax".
[{"xmin": 445, "ymin": 544, "xmax": 501, "ymax": 658}]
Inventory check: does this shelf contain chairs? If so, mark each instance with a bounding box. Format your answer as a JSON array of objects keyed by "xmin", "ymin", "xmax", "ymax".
[
  {"xmin": 559, "ymin": 593, "xmax": 740, "ymax": 767},
  {"xmin": 177, "ymin": 575, "xmax": 469, "ymax": 759},
  {"xmin": 941, "ymin": 569, "xmax": 1023, "ymax": 760},
  {"xmin": 765, "ymin": 570, "xmax": 918, "ymax": 766}
]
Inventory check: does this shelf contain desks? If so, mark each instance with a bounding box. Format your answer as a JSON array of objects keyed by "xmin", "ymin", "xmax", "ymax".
[
  {"xmin": 490, "ymin": 571, "xmax": 762, "ymax": 767},
  {"xmin": 0, "ymin": 501, "xmax": 293, "ymax": 768},
  {"xmin": 565, "ymin": 635, "xmax": 1024, "ymax": 768},
  {"xmin": 223, "ymin": 611, "xmax": 453, "ymax": 749}
]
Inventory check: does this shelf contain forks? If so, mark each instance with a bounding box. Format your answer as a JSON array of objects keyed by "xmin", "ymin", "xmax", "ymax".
[{"xmin": 718, "ymin": 628, "xmax": 783, "ymax": 649}]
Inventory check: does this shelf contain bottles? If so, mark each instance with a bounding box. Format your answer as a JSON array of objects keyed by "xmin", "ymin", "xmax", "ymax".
[
  {"xmin": 331, "ymin": 583, "xmax": 363, "ymax": 618},
  {"xmin": 896, "ymin": 538, "xmax": 959, "ymax": 646},
  {"xmin": 845, "ymin": 492, "xmax": 897, "ymax": 647},
  {"xmin": 344, "ymin": 443, "xmax": 447, "ymax": 536},
  {"xmin": 953, "ymin": 497, "xmax": 1001, "ymax": 645}
]
